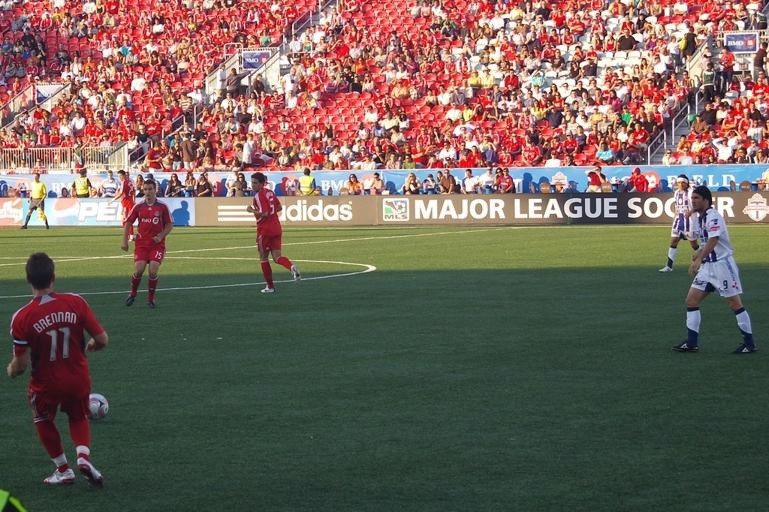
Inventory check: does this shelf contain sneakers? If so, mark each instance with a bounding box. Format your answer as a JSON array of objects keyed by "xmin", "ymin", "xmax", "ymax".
[
  {"xmin": 21, "ymin": 225, "xmax": 50, "ymax": 229},
  {"xmin": 43, "ymin": 468, "xmax": 76, "ymax": 485},
  {"xmin": 291, "ymin": 264, "xmax": 302, "ymax": 282},
  {"xmin": 146, "ymin": 299, "xmax": 157, "ymax": 308},
  {"xmin": 659, "ymin": 264, "xmax": 674, "ymax": 274},
  {"xmin": 126, "ymin": 294, "xmax": 136, "ymax": 306},
  {"xmin": 670, "ymin": 340, "xmax": 699, "ymax": 354},
  {"xmin": 733, "ymin": 342, "xmax": 757, "ymax": 356},
  {"xmin": 76, "ymin": 456, "xmax": 105, "ymax": 490},
  {"xmin": 259, "ymin": 286, "xmax": 275, "ymax": 294}
]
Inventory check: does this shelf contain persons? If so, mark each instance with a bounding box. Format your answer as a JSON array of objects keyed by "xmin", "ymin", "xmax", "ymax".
[
  {"xmin": 121, "ymin": 180, "xmax": 175, "ymax": 308},
  {"xmin": 658, "ymin": 174, "xmax": 700, "ymax": 273},
  {"xmin": 247, "ymin": 172, "xmax": 301, "ymax": 294},
  {"xmin": 111, "ymin": 170, "xmax": 136, "ymax": 241},
  {"xmin": 21, "ymin": 174, "xmax": 49, "ymax": 229},
  {"xmin": 0, "ymin": 489, "xmax": 28, "ymax": 512},
  {"xmin": 672, "ymin": 185, "xmax": 758, "ymax": 354},
  {"xmin": 7, "ymin": 251, "xmax": 109, "ymax": 488}
]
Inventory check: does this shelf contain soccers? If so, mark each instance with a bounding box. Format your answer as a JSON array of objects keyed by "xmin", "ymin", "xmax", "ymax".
[{"xmin": 88, "ymin": 394, "xmax": 108, "ymax": 419}]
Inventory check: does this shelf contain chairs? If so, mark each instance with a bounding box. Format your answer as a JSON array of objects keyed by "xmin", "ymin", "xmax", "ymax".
[{"xmin": 0, "ymin": 2, "xmax": 769, "ymax": 164}]
